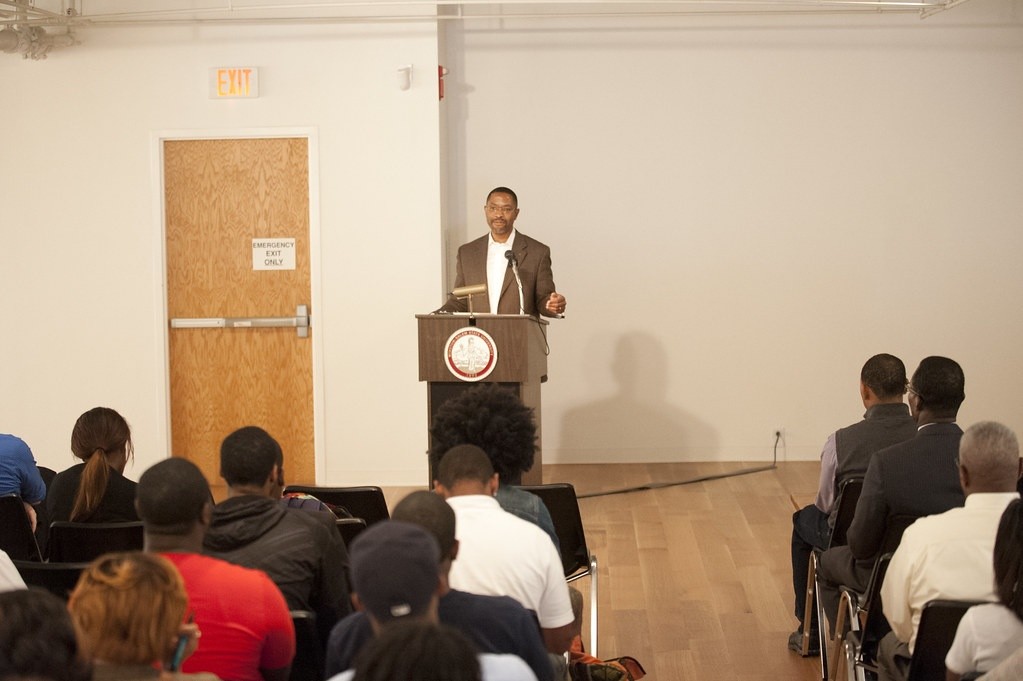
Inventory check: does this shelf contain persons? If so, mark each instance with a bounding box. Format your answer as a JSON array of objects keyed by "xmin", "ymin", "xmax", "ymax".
[
  {"xmin": 0, "ymin": 406, "xmax": 351, "ymax": 681},
  {"xmin": 323, "ymin": 382, "xmax": 585, "ymax": 680},
  {"xmin": 787, "ymin": 354, "xmax": 1023, "ymax": 680},
  {"xmin": 428, "ymin": 187, "xmax": 566, "ymax": 319}
]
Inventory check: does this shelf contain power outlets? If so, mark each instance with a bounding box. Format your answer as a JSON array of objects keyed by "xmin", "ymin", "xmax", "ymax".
[{"xmin": 771, "ymin": 428, "xmax": 785, "ymax": 446}]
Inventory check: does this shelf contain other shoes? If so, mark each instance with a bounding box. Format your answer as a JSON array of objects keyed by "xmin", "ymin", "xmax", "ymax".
[{"xmin": 787, "ymin": 630, "xmax": 820, "ymax": 656}]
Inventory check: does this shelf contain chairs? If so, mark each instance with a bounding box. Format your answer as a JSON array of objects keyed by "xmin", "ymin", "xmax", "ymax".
[
  {"xmin": 793, "ymin": 474, "xmax": 996, "ymax": 681},
  {"xmin": 0, "ymin": 468, "xmax": 597, "ymax": 681}
]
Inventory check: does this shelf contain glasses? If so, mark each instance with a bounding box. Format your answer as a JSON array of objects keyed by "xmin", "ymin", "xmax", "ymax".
[
  {"xmin": 952, "ymin": 454, "xmax": 970, "ymax": 477},
  {"xmin": 902, "ymin": 379, "xmax": 925, "ymax": 406},
  {"xmin": 485, "ymin": 202, "xmax": 517, "ymax": 215}
]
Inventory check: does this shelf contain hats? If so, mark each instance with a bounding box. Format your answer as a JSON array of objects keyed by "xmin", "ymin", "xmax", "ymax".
[{"xmin": 349, "ymin": 521, "xmax": 441, "ymax": 617}]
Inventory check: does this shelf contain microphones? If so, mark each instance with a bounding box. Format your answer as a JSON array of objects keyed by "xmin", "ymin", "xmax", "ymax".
[{"xmin": 505, "ymin": 250, "xmax": 517, "ymax": 266}]
